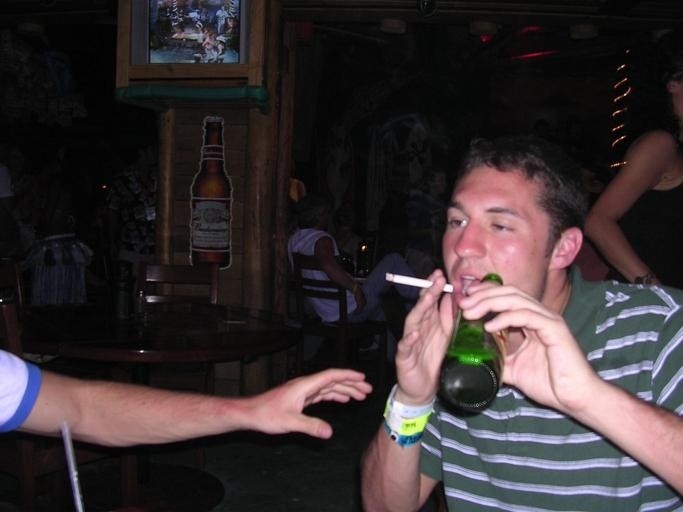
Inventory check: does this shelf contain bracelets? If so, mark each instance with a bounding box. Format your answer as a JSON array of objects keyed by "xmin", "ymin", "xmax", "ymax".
[{"xmin": 383, "ymin": 384, "xmax": 437, "ymax": 447}]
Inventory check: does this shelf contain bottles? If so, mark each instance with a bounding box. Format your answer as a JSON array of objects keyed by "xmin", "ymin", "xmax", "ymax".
[
  {"xmin": 439, "ymin": 272, "xmax": 506, "ymax": 418},
  {"xmin": 189, "ymin": 114, "xmax": 232, "ymax": 273},
  {"xmin": 118, "ymin": 282, "xmax": 147, "ymax": 321}
]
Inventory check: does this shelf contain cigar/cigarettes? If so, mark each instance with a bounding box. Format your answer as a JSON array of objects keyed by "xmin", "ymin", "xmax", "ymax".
[{"xmin": 222, "ymin": 319, "xmax": 247, "ymax": 325}]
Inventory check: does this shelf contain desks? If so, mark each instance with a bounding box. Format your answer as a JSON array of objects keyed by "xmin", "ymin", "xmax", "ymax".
[{"xmin": 21, "ymin": 303, "xmax": 299, "ymax": 509}]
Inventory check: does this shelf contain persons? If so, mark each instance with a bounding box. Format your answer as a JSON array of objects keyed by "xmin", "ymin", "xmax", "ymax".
[
  {"xmin": 0, "ymin": 350, "xmax": 373, "ymax": 447},
  {"xmin": 0, "ymin": 124, "xmax": 159, "ymax": 296},
  {"xmin": 289, "ymin": 110, "xmax": 508, "ymax": 382},
  {"xmin": 507, "ymin": 31, "xmax": 681, "ymax": 290},
  {"xmin": 363, "ymin": 139, "xmax": 683, "ymax": 512}
]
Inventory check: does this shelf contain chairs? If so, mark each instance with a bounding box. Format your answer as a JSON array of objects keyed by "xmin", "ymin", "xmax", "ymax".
[
  {"xmin": 288, "ymin": 249, "xmax": 393, "ymax": 384},
  {"xmin": 0, "ymin": 249, "xmax": 219, "ymax": 511}
]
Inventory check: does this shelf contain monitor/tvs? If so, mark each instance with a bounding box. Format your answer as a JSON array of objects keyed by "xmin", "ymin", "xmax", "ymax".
[{"xmin": 131, "ymin": 0, "xmax": 250, "ymax": 65}]
